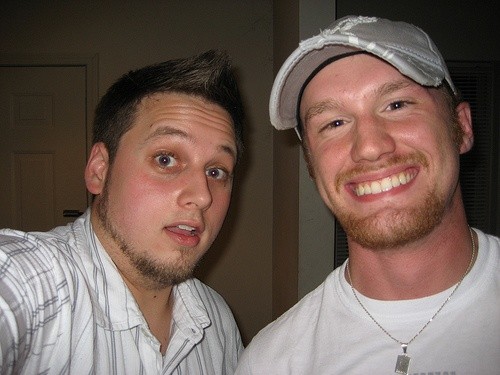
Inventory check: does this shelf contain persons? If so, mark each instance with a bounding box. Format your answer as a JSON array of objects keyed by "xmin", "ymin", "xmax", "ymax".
[
  {"xmin": 231, "ymin": 16, "xmax": 500, "ymax": 375},
  {"xmin": 0, "ymin": 49, "xmax": 246, "ymax": 375}
]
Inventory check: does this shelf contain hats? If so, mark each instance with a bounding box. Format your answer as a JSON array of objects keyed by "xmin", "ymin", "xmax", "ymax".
[{"xmin": 267, "ymin": 14, "xmax": 456, "ymax": 145}]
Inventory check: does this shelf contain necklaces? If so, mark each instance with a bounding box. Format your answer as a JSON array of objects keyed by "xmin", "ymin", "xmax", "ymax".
[{"xmin": 346, "ymin": 223, "xmax": 477, "ymax": 375}]
